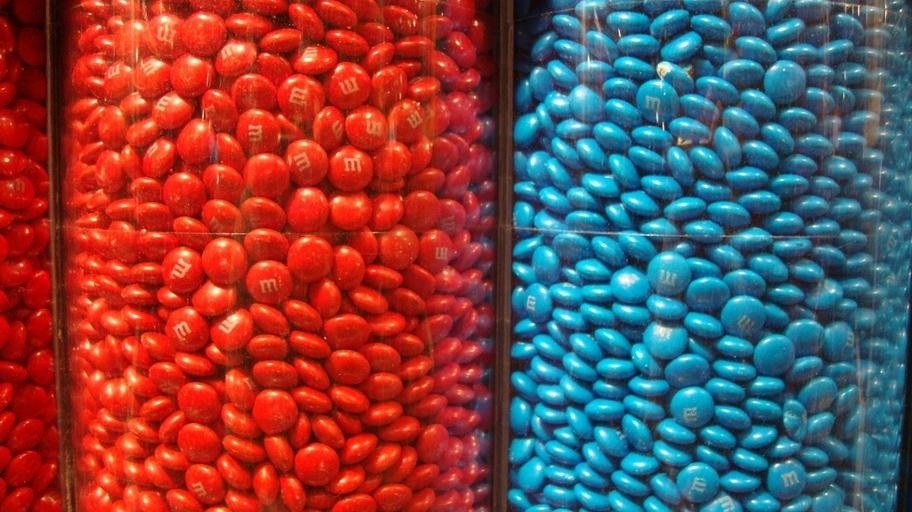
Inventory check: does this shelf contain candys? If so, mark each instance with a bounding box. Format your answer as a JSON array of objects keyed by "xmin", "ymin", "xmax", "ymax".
[
  {"xmin": 1, "ymin": 1, "xmax": 65, "ymax": 511},
  {"xmin": 65, "ymin": 0, "xmax": 912, "ymax": 512}
]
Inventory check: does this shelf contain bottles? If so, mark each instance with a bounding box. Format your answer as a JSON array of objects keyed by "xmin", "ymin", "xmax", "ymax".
[
  {"xmin": 59, "ymin": 0, "xmax": 495, "ymax": 512},
  {"xmin": 509, "ymin": 1, "xmax": 910, "ymax": 511}
]
